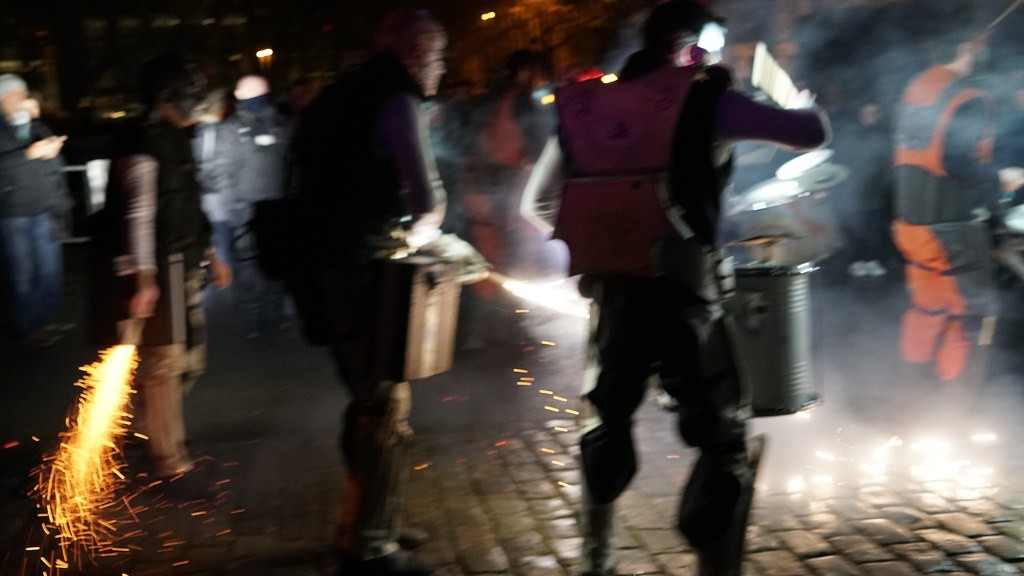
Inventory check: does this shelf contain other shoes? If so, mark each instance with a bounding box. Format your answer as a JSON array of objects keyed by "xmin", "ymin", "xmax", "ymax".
[
  {"xmin": 158, "ymin": 473, "xmax": 210, "ymax": 499},
  {"xmin": 345, "ymin": 549, "xmax": 435, "ymax": 576},
  {"xmin": 398, "ymin": 527, "xmax": 429, "ymax": 549}
]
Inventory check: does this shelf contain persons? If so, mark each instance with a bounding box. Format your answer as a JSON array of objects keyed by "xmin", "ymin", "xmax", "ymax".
[{"xmin": 0, "ymin": 0, "xmax": 1024, "ymax": 576}]
